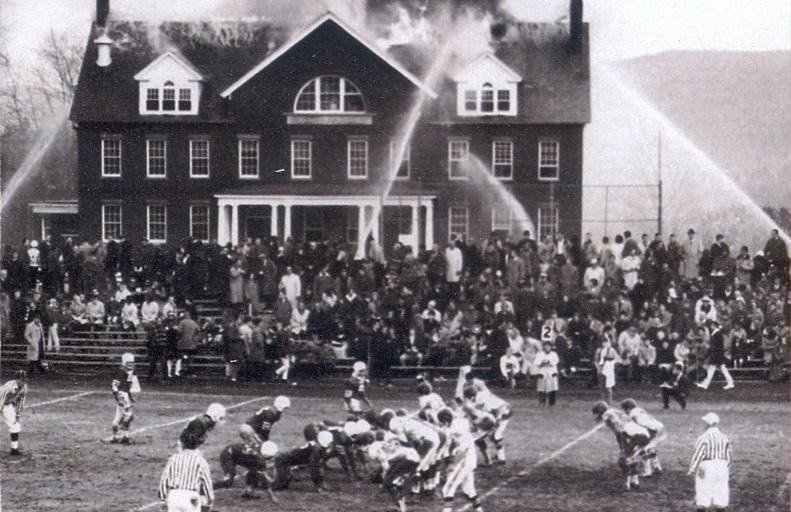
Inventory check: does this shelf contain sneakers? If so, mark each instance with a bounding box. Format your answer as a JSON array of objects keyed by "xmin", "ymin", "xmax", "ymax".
[
  {"xmin": 242, "ymin": 489, "xmax": 261, "ymax": 499},
  {"xmin": 406, "ymin": 494, "xmax": 420, "ymax": 503}
]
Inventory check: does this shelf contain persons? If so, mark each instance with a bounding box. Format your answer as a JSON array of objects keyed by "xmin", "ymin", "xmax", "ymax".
[
  {"xmin": 589, "ymin": 398, "xmax": 734, "ymax": 512},
  {"xmin": 105, "ymin": 354, "xmax": 511, "ymax": 512},
  {"xmin": 0, "ymin": 370, "xmax": 31, "ymax": 455},
  {"xmin": 0, "ymin": 230, "xmax": 790, "ymax": 406}
]
